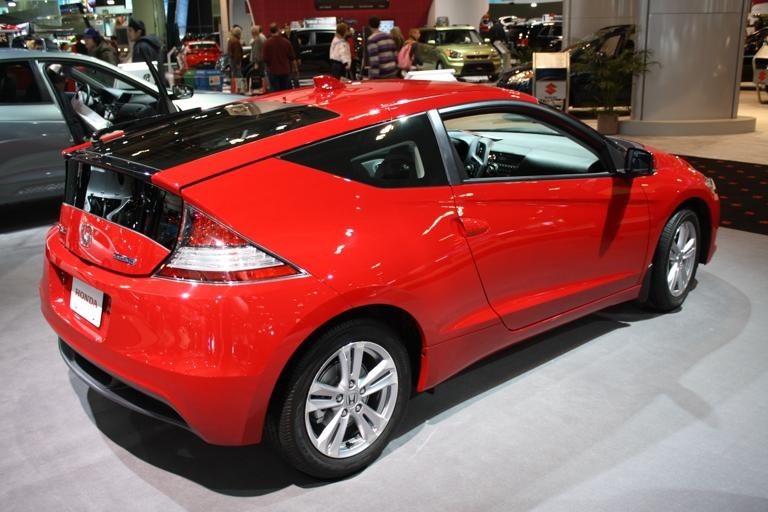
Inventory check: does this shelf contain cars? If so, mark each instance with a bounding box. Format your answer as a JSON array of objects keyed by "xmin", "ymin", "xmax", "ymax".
[
  {"xmin": 0, "ymin": 46, "xmax": 262, "ymax": 207},
  {"xmin": 413, "ymin": 24, "xmax": 501, "ymax": 81},
  {"xmin": 224, "ymin": 26, "xmax": 366, "ymax": 89},
  {"xmin": 37, "ymin": 74, "xmax": 720, "ymax": 483},
  {"xmin": 494, "ymin": 14, "xmax": 639, "ymax": 106},
  {"xmin": 176, "ymin": 41, "xmax": 223, "ymax": 69}
]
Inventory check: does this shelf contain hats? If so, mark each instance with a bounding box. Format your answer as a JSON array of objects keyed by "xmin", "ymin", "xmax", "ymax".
[{"xmin": 80, "ymin": 28, "xmax": 95, "ymax": 37}]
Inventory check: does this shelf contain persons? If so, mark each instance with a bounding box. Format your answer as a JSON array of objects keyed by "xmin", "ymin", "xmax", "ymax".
[
  {"xmin": 346, "ymin": 25, "xmax": 357, "ymax": 79},
  {"xmin": 367, "ymin": 16, "xmax": 400, "ymax": 80},
  {"xmin": 479, "ymin": 14, "xmax": 490, "ymax": 37},
  {"xmin": 16, "ymin": 19, "xmax": 163, "ymax": 66},
  {"xmin": 390, "ymin": 26, "xmax": 404, "ymax": 46},
  {"xmin": 489, "ymin": 15, "xmax": 505, "ymax": 39},
  {"xmin": 228, "ymin": 22, "xmax": 304, "ymax": 92},
  {"xmin": 399, "ymin": 28, "xmax": 421, "ymax": 80},
  {"xmin": 329, "ymin": 21, "xmax": 352, "ymax": 80}
]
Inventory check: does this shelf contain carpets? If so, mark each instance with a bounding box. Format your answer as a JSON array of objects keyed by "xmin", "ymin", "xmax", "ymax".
[{"xmin": 673, "ymin": 155, "xmax": 768, "ymax": 235}]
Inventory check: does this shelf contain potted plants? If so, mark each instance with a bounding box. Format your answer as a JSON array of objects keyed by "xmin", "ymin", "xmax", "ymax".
[{"xmin": 571, "ymin": 46, "xmax": 664, "ymax": 135}]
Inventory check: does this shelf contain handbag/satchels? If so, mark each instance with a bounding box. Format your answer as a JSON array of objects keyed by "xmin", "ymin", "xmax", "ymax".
[
  {"xmin": 249, "ymin": 75, "xmax": 267, "ymax": 96},
  {"xmin": 231, "ymin": 78, "xmax": 248, "ymax": 94},
  {"xmin": 398, "ymin": 42, "xmax": 412, "ymax": 70}
]
